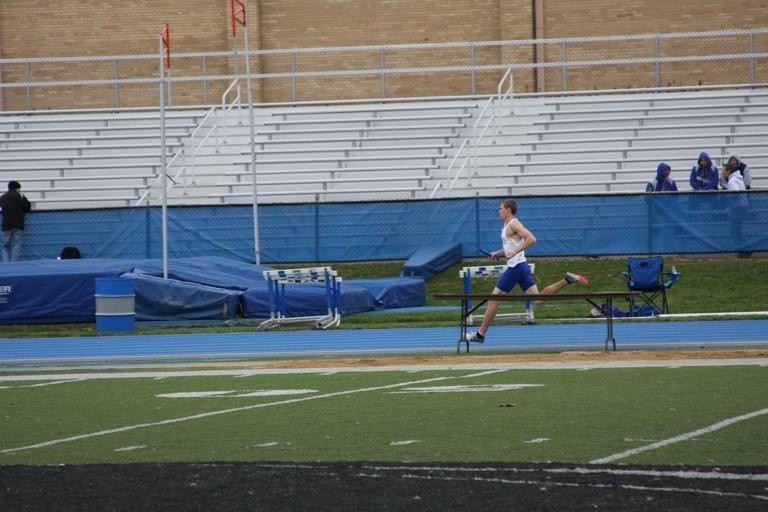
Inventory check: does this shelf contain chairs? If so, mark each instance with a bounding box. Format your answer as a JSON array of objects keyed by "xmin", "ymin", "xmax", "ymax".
[{"xmin": 623, "ymin": 253, "xmax": 683, "ymax": 312}]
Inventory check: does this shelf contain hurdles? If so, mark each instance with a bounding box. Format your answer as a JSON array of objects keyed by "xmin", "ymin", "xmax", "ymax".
[
  {"xmin": 459, "ymin": 263, "xmax": 536, "ymax": 324},
  {"xmin": 260, "ymin": 265, "xmax": 343, "ymax": 330}
]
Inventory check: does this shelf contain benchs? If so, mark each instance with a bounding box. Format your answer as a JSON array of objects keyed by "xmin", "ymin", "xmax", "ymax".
[
  {"xmin": 0, "ymin": 83, "xmax": 767, "ymax": 214},
  {"xmin": 430, "ymin": 289, "xmax": 642, "ymax": 353}
]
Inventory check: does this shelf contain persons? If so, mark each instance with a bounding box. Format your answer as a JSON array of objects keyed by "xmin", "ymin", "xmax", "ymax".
[
  {"xmin": 719, "ymin": 164, "xmax": 755, "ymax": 258},
  {"xmin": 0, "ymin": 180, "xmax": 30, "ymax": 262},
  {"xmin": 721, "ymin": 152, "xmax": 751, "ymax": 189},
  {"xmin": 687, "ymin": 151, "xmax": 718, "ymax": 209},
  {"xmin": 463, "ymin": 199, "xmax": 589, "ymax": 343},
  {"xmin": 644, "ymin": 162, "xmax": 683, "ymax": 250}
]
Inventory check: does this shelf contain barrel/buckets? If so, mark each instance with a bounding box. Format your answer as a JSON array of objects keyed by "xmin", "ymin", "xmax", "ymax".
[{"xmin": 94, "ymin": 276, "xmax": 137, "ymax": 333}]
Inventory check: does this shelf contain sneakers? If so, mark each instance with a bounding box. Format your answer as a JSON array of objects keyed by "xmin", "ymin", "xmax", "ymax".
[
  {"xmin": 565, "ymin": 272, "xmax": 589, "ymax": 286},
  {"xmin": 462, "ymin": 331, "xmax": 485, "ymax": 343}
]
